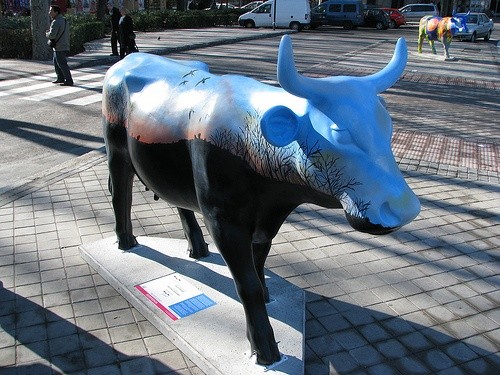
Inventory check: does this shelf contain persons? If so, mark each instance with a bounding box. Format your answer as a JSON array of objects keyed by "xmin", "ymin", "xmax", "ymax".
[
  {"xmin": 118, "ymin": 7, "xmax": 139, "ymax": 61},
  {"xmin": 44, "ymin": 5, "xmax": 74, "ymax": 86},
  {"xmin": 105, "ymin": 3, "xmax": 122, "ymax": 56}
]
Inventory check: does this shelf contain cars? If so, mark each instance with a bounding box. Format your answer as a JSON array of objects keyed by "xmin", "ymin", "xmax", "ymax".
[
  {"xmin": 204, "ymin": 1, "xmax": 263, "ymax": 12},
  {"xmin": 451, "ymin": 12, "xmax": 494, "ymax": 42},
  {"xmin": 360, "ymin": 9, "xmax": 391, "ymax": 30},
  {"xmin": 378, "ymin": 8, "xmax": 406, "ymax": 29}
]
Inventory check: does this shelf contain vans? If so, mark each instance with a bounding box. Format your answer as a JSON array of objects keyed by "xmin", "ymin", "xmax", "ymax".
[{"xmin": 304, "ymin": 0, "xmax": 364, "ymax": 30}]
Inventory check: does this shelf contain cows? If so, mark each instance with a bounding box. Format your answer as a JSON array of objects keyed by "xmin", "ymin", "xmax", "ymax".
[
  {"xmin": 102, "ymin": 35, "xmax": 422, "ymax": 365},
  {"xmin": 418, "ymin": 11, "xmax": 470, "ymax": 58}
]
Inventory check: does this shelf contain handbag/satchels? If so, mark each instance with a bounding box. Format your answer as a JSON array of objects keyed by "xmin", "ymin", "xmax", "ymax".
[{"xmin": 47, "ymin": 39, "xmax": 57, "ymax": 48}]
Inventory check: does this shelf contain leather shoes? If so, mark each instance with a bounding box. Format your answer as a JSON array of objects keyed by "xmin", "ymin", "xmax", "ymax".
[
  {"xmin": 60, "ymin": 83, "xmax": 73, "ymax": 86},
  {"xmin": 52, "ymin": 79, "xmax": 65, "ymax": 84}
]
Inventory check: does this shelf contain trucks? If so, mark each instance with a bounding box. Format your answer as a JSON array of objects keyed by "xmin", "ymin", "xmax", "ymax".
[{"xmin": 238, "ymin": 0, "xmax": 312, "ymax": 32}]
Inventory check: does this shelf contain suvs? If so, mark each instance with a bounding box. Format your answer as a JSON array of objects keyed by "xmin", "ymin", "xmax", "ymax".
[{"xmin": 390, "ymin": 3, "xmax": 439, "ymax": 22}]
